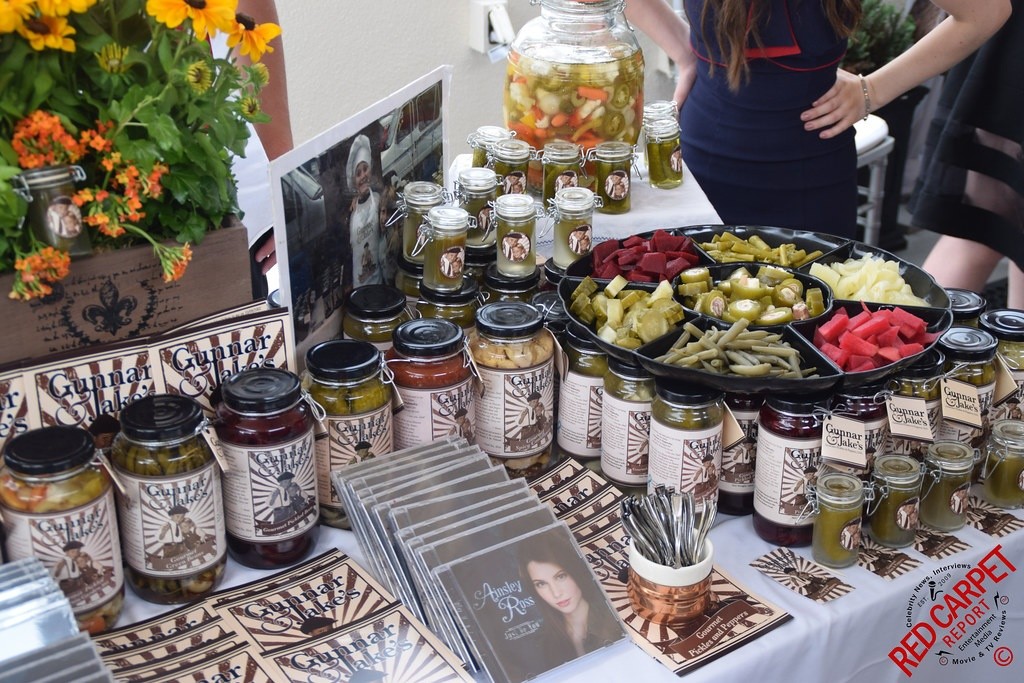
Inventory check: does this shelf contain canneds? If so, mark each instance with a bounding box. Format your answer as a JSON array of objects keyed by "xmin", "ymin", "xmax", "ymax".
[
  {"xmin": 0, "ymin": 247, "xmax": 571, "ymax": 637},
  {"xmin": 554, "ymin": 288, "xmax": 1024, "ymax": 570},
  {"xmin": 384, "ymin": 100, "xmax": 684, "ymax": 292},
  {"xmin": 10, "ymin": 163, "xmax": 96, "ymax": 259}
]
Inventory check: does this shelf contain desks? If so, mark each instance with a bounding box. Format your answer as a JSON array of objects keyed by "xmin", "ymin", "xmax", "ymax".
[{"xmin": 0, "ymin": 108, "xmax": 1024, "ymax": 683}]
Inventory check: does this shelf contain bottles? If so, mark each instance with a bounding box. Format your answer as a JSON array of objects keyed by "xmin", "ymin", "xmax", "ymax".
[
  {"xmin": 342, "ymin": 102, "xmax": 682, "ymax": 292},
  {"xmin": 504, "ymin": 0, "xmax": 644, "ymax": 193},
  {"xmin": 12, "ymin": 165, "xmax": 94, "ymax": 259},
  {"xmin": 796, "ymin": 421, "xmax": 1018, "ymax": 567}
]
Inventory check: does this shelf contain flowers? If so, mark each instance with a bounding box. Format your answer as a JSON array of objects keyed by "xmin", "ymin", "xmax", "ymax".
[{"xmin": 0, "ymin": 0, "xmax": 287, "ymax": 303}]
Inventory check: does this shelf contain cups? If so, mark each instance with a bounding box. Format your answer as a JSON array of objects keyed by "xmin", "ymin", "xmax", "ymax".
[{"xmin": 627, "ymin": 529, "xmax": 713, "ymax": 626}]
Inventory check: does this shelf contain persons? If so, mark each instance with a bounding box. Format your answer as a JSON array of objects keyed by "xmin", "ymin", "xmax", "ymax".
[
  {"xmin": 568, "ymin": 0, "xmax": 1013, "ymax": 241},
  {"xmin": 907, "ymin": 0, "xmax": 1024, "ymax": 310},
  {"xmin": 345, "ymin": 134, "xmax": 404, "ymax": 288},
  {"xmin": 516, "ymin": 528, "xmax": 623, "ymax": 671}
]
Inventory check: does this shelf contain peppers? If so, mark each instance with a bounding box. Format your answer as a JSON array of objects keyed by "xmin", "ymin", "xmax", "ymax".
[{"xmin": 677, "ymin": 265, "xmax": 824, "ymax": 325}]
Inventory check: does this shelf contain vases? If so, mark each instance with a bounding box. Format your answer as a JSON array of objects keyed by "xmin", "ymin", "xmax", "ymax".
[{"xmin": 0, "ymin": 213, "xmax": 253, "ymax": 368}]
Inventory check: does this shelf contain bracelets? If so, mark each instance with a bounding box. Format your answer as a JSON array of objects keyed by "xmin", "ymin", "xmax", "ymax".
[{"xmin": 858, "ymin": 74, "xmax": 870, "ymax": 121}]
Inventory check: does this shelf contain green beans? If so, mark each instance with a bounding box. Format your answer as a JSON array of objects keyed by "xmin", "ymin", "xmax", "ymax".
[{"xmin": 697, "ymin": 231, "xmax": 825, "ymax": 269}]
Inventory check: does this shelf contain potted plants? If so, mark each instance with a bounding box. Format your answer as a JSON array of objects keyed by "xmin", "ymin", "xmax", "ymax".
[{"xmin": 845, "ymin": 0, "xmax": 932, "ymax": 252}]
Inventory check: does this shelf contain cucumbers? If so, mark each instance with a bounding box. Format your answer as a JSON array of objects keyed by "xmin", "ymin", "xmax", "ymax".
[{"xmin": 570, "ymin": 275, "xmax": 685, "ymax": 351}]
